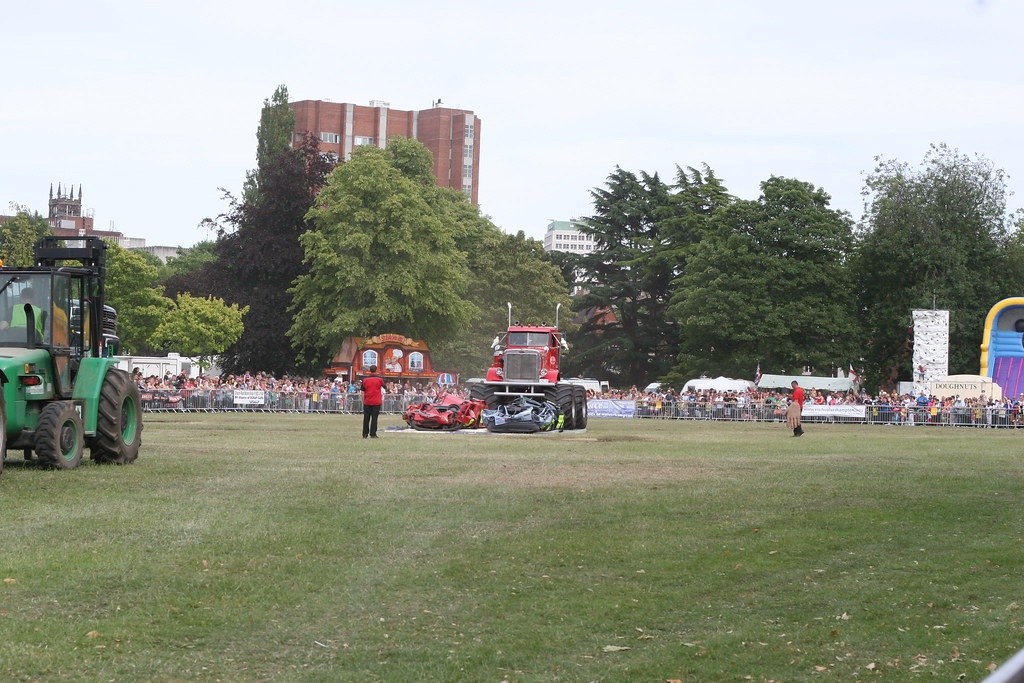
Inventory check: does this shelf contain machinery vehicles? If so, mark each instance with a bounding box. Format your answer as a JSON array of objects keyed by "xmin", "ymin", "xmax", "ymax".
[
  {"xmin": 0, "ymin": 232, "xmax": 145, "ymax": 473},
  {"xmin": 464, "ymin": 300, "xmax": 588, "ymax": 431}
]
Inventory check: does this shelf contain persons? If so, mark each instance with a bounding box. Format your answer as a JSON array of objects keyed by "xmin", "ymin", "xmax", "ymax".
[
  {"xmin": 785, "ymin": 381, "xmax": 805, "ymax": 437},
  {"xmin": 359, "ymin": 364, "xmax": 387, "ymax": 438},
  {"xmin": 0, "ymin": 287, "xmax": 48, "ymax": 347},
  {"xmin": 585, "ymin": 383, "xmax": 1024, "ymax": 428},
  {"xmin": 126, "ymin": 367, "xmax": 471, "ymax": 423}
]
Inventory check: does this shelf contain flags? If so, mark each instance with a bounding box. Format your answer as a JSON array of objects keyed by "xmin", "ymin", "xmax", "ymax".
[
  {"xmin": 845, "ymin": 363, "xmax": 860, "ymax": 395},
  {"xmin": 754, "ymin": 362, "xmax": 762, "ymax": 385}
]
